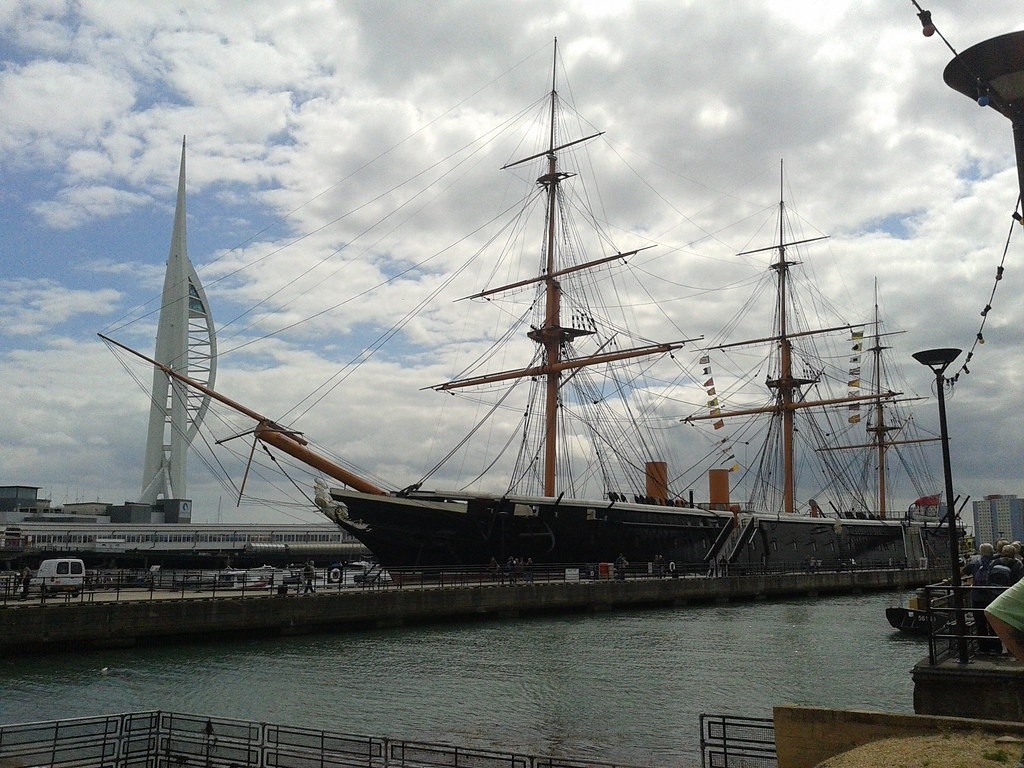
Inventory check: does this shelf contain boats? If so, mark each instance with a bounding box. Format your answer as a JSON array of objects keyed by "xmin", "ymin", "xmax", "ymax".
[
  {"xmin": 885, "ymin": 579, "xmax": 972, "ymax": 633},
  {"xmin": 282, "ymin": 570, "xmax": 302, "ymax": 582},
  {"xmin": 213, "ymin": 567, "xmax": 267, "ymax": 587},
  {"xmin": 347, "ymin": 560, "xmax": 369, "ymax": 570}
]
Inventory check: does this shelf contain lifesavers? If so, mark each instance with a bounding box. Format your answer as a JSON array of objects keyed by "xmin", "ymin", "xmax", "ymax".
[
  {"xmin": 330, "ymin": 568, "xmax": 341, "ymax": 582},
  {"xmin": 669, "ymin": 562, "xmax": 676, "ymax": 571}
]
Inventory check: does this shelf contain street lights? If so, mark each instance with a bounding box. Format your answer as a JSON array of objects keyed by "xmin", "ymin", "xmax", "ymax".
[
  {"xmin": 912, "ymin": 348, "xmax": 974, "ymax": 665},
  {"xmin": 943, "ymin": 29, "xmax": 1024, "ymax": 217}
]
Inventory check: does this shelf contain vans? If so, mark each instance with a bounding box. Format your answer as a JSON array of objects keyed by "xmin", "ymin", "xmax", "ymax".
[{"xmin": 36, "ymin": 559, "xmax": 85, "ymax": 597}]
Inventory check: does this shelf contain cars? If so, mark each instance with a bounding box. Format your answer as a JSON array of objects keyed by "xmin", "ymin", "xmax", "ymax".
[{"xmin": 17, "ymin": 579, "xmax": 42, "ymax": 596}]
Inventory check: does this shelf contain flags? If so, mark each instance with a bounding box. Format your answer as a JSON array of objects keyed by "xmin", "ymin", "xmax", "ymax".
[
  {"xmin": 852, "ymin": 331, "xmax": 864, "ymax": 338},
  {"xmin": 851, "ymin": 343, "xmax": 862, "ymax": 351},
  {"xmin": 849, "ymin": 403, "xmax": 860, "ymax": 410},
  {"xmin": 848, "ymin": 379, "xmax": 859, "ymax": 387},
  {"xmin": 698, "ymin": 355, "xmax": 724, "ymax": 430},
  {"xmin": 849, "ymin": 367, "xmax": 860, "ymax": 375},
  {"xmin": 848, "ymin": 391, "xmax": 859, "ymax": 397},
  {"xmin": 848, "ymin": 414, "xmax": 860, "ymax": 423},
  {"xmin": 917, "ymin": 494, "xmax": 941, "ymax": 506},
  {"xmin": 850, "ymin": 355, "xmax": 862, "ymax": 363}
]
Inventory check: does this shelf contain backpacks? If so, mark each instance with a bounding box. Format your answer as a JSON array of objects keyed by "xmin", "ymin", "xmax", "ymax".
[
  {"xmin": 975, "ymin": 557, "xmax": 995, "ymax": 586},
  {"xmin": 991, "ymin": 558, "xmax": 1021, "ymax": 587}
]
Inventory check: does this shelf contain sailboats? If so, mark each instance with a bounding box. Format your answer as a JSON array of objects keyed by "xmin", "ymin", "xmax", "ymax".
[{"xmin": 98, "ymin": 36, "xmax": 968, "ymax": 582}]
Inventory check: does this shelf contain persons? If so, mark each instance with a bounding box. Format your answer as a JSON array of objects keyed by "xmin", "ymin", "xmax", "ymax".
[
  {"xmin": 984, "ymin": 576, "xmax": 1024, "ymax": 659},
  {"xmin": 946, "ymin": 540, "xmax": 1024, "ymax": 652},
  {"xmin": 805, "ymin": 555, "xmax": 816, "ymax": 572},
  {"xmin": 654, "ymin": 555, "xmax": 664, "ymax": 578},
  {"xmin": 487, "ymin": 556, "xmax": 534, "ymax": 583},
  {"xmin": 15, "ymin": 568, "xmax": 32, "ymax": 597},
  {"xmin": 299, "ymin": 560, "xmax": 316, "ymax": 593},
  {"xmin": 616, "ymin": 553, "xmax": 629, "ymax": 580},
  {"xmin": 709, "ymin": 555, "xmax": 727, "ymax": 577}
]
